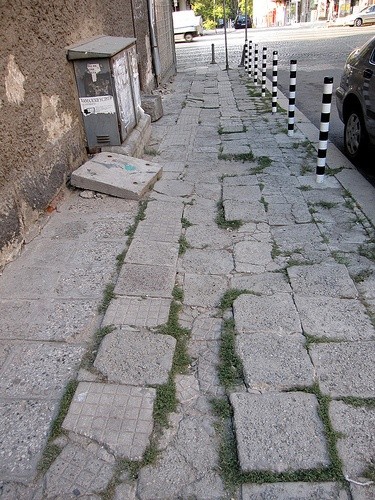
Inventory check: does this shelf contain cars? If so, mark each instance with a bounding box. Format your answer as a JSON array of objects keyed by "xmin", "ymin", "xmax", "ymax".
[
  {"xmin": 340, "ymin": 3, "xmax": 375, "ymax": 27},
  {"xmin": 334, "ymin": 34, "xmax": 375, "ymax": 154},
  {"xmin": 234, "ymin": 15, "xmax": 252, "ymax": 29}
]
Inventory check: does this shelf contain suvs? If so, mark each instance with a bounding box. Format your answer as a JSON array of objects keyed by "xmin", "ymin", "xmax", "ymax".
[{"xmin": 172, "ymin": 10, "xmax": 204, "ymax": 42}]
